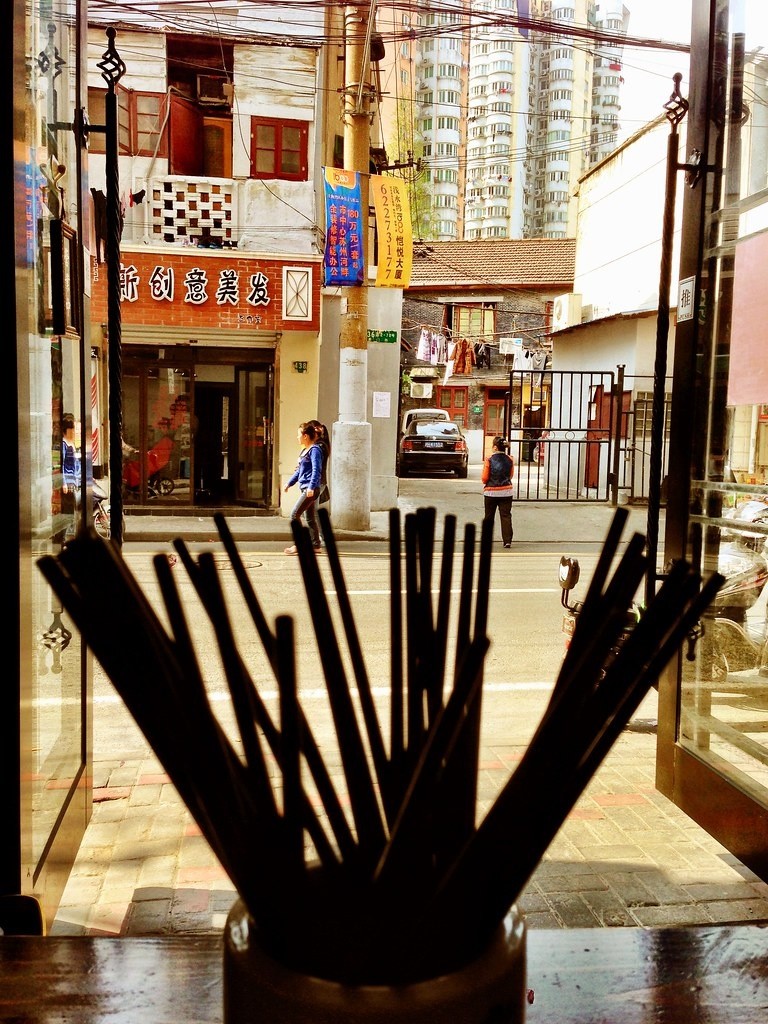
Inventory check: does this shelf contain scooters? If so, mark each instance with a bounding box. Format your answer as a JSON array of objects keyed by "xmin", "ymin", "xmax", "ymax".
[
  {"xmin": 121, "ymin": 448, "xmax": 175, "ymax": 496},
  {"xmin": 559, "ymin": 555, "xmax": 646, "ymax": 691},
  {"xmin": 93, "ymin": 476, "xmax": 125, "ymax": 544}
]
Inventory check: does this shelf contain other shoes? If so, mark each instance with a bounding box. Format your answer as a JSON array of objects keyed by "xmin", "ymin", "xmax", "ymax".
[
  {"xmin": 313, "ymin": 547, "xmax": 322, "ymax": 554},
  {"xmin": 503, "ymin": 542, "xmax": 511, "ymax": 548},
  {"xmin": 284, "ymin": 545, "xmax": 298, "ymax": 554}
]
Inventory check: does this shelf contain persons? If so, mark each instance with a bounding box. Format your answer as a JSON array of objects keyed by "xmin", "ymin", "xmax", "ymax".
[
  {"xmin": 283, "ymin": 423, "xmax": 321, "ymax": 554},
  {"xmin": 481, "ymin": 436, "xmax": 514, "ymax": 548},
  {"xmin": 62, "ymin": 413, "xmax": 77, "ymax": 515},
  {"xmin": 308, "ymin": 420, "xmax": 330, "ymax": 512}
]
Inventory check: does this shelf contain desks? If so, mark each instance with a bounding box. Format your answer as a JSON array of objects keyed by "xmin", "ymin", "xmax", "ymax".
[{"xmin": 0, "ymin": 919, "xmax": 768, "ymax": 1024}]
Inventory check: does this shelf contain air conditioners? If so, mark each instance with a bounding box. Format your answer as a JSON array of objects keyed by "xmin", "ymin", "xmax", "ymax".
[
  {"xmin": 410, "ymin": 383, "xmax": 433, "ymax": 399},
  {"xmin": 499, "ymin": 337, "xmax": 523, "ymax": 355},
  {"xmin": 553, "ymin": 292, "xmax": 581, "ymax": 331}
]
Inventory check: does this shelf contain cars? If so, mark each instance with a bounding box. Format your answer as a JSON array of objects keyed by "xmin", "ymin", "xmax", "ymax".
[
  {"xmin": 532, "ymin": 430, "xmax": 549, "ymax": 467},
  {"xmin": 397, "ymin": 409, "xmax": 451, "ymax": 442},
  {"xmin": 397, "ymin": 419, "xmax": 470, "ymax": 479}
]
